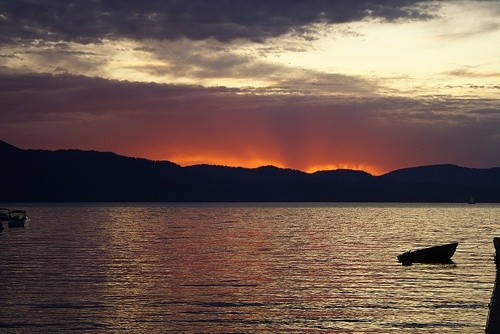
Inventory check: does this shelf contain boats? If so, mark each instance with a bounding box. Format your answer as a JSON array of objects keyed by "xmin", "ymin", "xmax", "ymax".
[
  {"xmin": 397, "ymin": 242, "xmax": 459, "ymax": 263},
  {"xmin": 0, "ymin": 208, "xmax": 10, "ymax": 220},
  {"xmin": 8, "ymin": 210, "xmax": 30, "ymax": 227}
]
